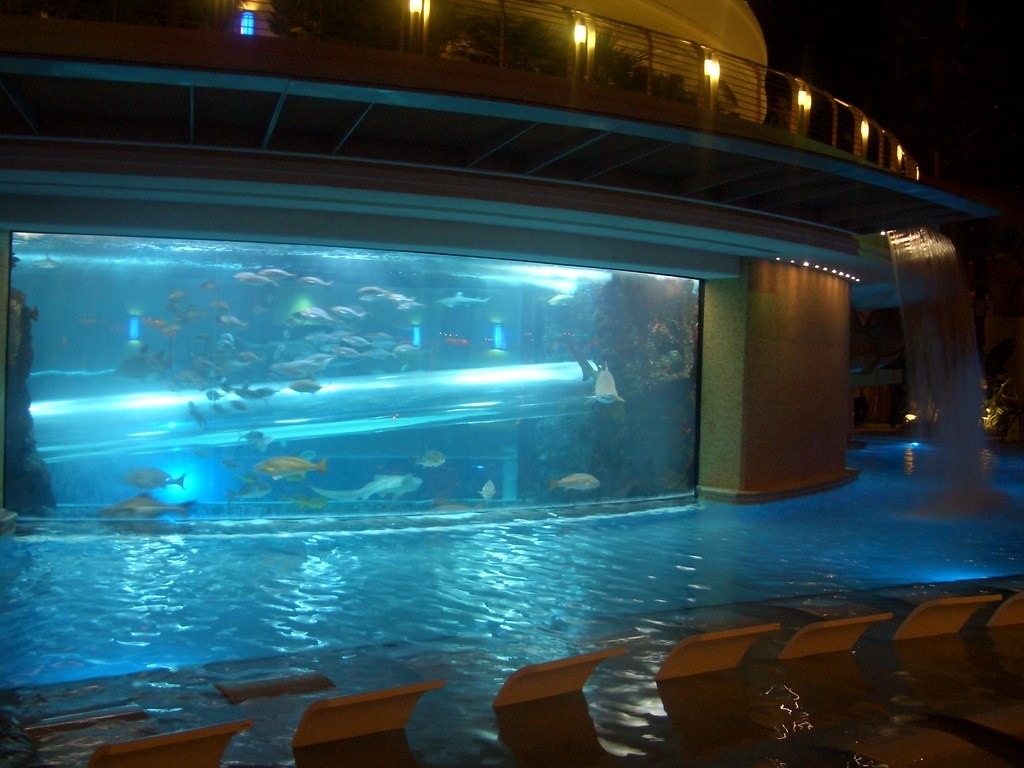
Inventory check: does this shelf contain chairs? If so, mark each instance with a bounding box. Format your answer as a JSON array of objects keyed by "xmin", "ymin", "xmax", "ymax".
[{"xmin": 1, "ymin": 575, "xmax": 1024, "ymax": 767}]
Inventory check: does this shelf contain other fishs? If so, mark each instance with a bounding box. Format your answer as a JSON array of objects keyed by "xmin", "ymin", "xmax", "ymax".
[{"xmin": 32, "ymin": 258, "xmax": 627, "ymax": 518}]
